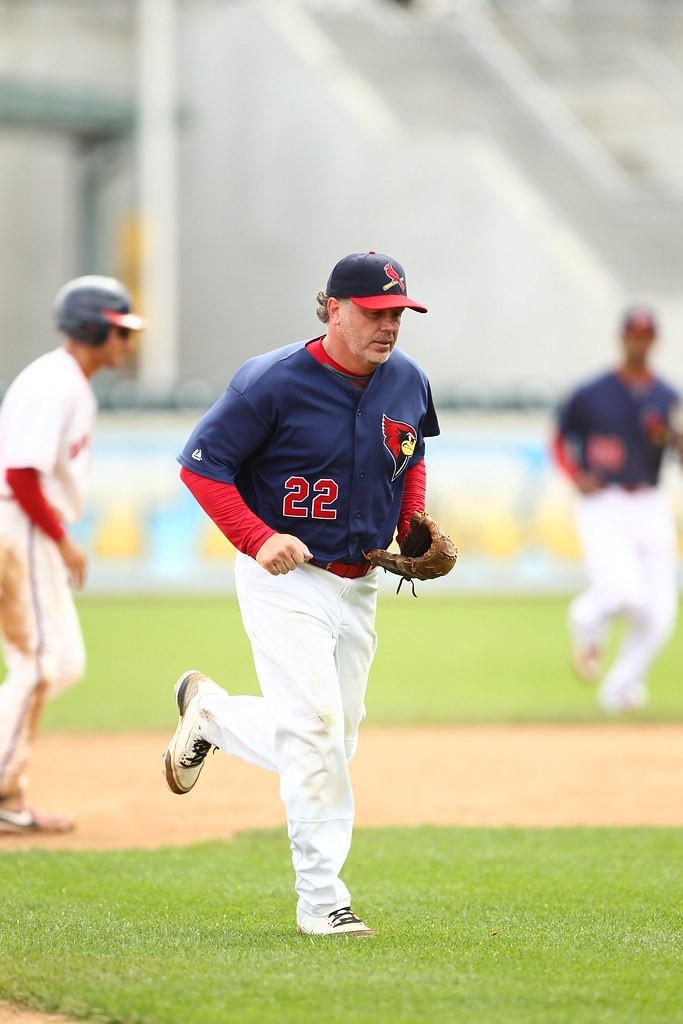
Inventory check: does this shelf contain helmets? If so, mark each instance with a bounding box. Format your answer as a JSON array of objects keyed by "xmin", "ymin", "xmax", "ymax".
[{"xmin": 52, "ymin": 275, "xmax": 144, "ymax": 346}]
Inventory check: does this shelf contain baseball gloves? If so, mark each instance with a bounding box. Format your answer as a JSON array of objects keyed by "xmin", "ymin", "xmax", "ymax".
[{"xmin": 361, "ymin": 511, "xmax": 458, "ymax": 598}]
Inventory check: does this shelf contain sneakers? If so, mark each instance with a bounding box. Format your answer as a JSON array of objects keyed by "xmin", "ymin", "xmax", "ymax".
[
  {"xmin": 296, "ymin": 903, "xmax": 373, "ymax": 935},
  {"xmin": 0, "ymin": 807, "xmax": 78, "ymax": 835},
  {"xmin": 162, "ymin": 671, "xmax": 227, "ymax": 796}
]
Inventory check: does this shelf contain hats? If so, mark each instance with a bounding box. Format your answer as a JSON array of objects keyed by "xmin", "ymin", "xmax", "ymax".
[
  {"xmin": 326, "ymin": 251, "xmax": 428, "ymax": 313},
  {"xmin": 623, "ymin": 311, "xmax": 655, "ymax": 340}
]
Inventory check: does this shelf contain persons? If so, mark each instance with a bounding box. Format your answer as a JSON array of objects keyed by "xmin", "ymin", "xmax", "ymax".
[
  {"xmin": 0, "ymin": 275, "xmax": 145, "ymax": 839},
  {"xmin": 165, "ymin": 252, "xmax": 440, "ymax": 938},
  {"xmin": 550, "ymin": 305, "xmax": 683, "ymax": 712}
]
